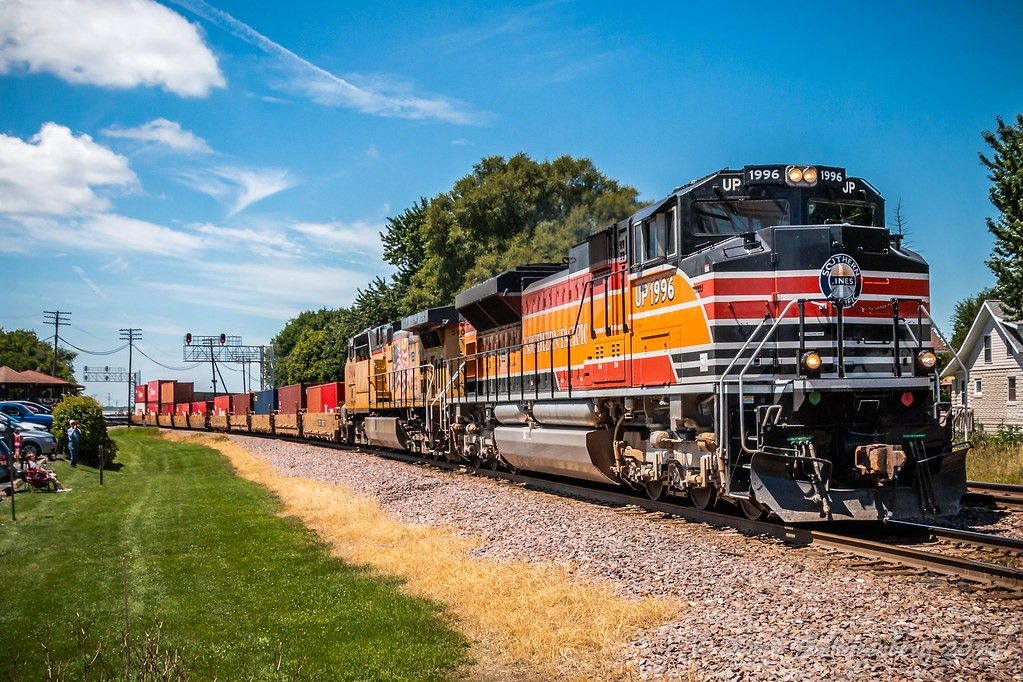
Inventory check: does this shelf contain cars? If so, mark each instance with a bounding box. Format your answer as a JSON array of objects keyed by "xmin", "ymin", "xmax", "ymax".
[{"xmin": 0, "ymin": 400, "xmax": 58, "ymax": 482}]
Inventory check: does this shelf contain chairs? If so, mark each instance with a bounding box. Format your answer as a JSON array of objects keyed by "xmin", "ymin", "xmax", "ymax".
[{"xmin": 16, "ymin": 470, "xmax": 51, "ymax": 494}]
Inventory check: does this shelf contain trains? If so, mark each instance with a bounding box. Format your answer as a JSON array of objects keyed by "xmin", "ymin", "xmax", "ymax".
[{"xmin": 133, "ymin": 164, "xmax": 977, "ymax": 529}]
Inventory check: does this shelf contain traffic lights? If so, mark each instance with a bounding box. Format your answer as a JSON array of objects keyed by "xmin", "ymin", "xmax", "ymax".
[
  {"xmin": 220, "ymin": 333, "xmax": 226, "ymax": 344},
  {"xmin": 186, "ymin": 332, "xmax": 192, "ymax": 343}
]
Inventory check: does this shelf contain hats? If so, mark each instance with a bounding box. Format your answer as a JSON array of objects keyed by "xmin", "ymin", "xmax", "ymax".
[{"xmin": 70, "ymin": 420, "xmax": 75, "ymax": 423}]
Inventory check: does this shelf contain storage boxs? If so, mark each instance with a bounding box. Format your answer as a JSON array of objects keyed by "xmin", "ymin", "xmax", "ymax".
[{"xmin": 133, "ymin": 379, "xmax": 346, "ymax": 417}]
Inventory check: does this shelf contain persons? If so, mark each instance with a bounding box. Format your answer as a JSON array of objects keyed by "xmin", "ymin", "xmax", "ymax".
[{"xmin": 12, "ymin": 419, "xmax": 82, "ymax": 493}]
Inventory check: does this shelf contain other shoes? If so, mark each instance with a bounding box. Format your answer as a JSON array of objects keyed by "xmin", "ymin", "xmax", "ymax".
[
  {"xmin": 62, "ymin": 487, "xmax": 72, "ymax": 491},
  {"xmin": 53, "ymin": 488, "xmax": 64, "ymax": 492}
]
